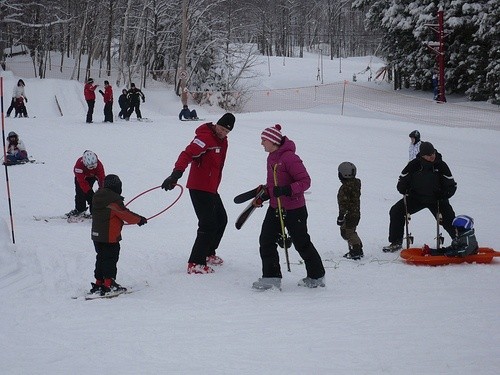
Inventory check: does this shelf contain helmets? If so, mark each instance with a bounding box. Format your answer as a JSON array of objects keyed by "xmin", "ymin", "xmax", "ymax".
[
  {"xmin": 338, "ymin": 161, "xmax": 356, "ymax": 180},
  {"xmin": 8, "ymin": 132, "xmax": 17, "ymax": 138},
  {"xmin": 452, "ymin": 215, "xmax": 474, "ymax": 229}
]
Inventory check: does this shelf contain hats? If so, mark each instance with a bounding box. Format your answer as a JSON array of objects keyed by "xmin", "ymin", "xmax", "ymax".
[
  {"xmin": 409, "ymin": 129, "xmax": 420, "ymax": 145},
  {"xmin": 87, "ymin": 78, "xmax": 94, "ymax": 84},
  {"xmin": 261, "ymin": 124, "xmax": 283, "ymax": 145},
  {"xmin": 83, "ymin": 152, "xmax": 97, "ymax": 166},
  {"xmin": 131, "ymin": 83, "xmax": 135, "ymax": 87},
  {"xmin": 420, "ymin": 142, "xmax": 434, "ymax": 154},
  {"xmin": 216, "ymin": 113, "xmax": 235, "ymax": 131},
  {"xmin": 103, "ymin": 174, "xmax": 121, "ymax": 189},
  {"xmin": 424, "ymin": 149, "xmax": 437, "ymax": 157}
]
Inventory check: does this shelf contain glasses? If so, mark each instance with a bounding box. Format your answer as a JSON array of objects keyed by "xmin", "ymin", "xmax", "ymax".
[{"xmin": 86, "ymin": 164, "xmax": 97, "ymax": 169}]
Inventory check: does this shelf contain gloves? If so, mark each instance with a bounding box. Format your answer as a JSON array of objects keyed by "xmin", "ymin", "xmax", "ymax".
[
  {"xmin": 444, "ymin": 250, "xmax": 458, "ymax": 258},
  {"xmin": 137, "ymin": 217, "xmax": 148, "ymax": 227},
  {"xmin": 435, "ymin": 247, "xmax": 446, "ymax": 254},
  {"xmin": 399, "ymin": 181, "xmax": 410, "ymax": 194},
  {"xmin": 162, "ymin": 170, "xmax": 182, "ymax": 191},
  {"xmin": 337, "ymin": 216, "xmax": 344, "ymax": 226},
  {"xmin": 273, "ymin": 186, "xmax": 292, "ymax": 197},
  {"xmin": 434, "ymin": 189, "xmax": 443, "ymax": 198},
  {"xmin": 99, "ymin": 90, "xmax": 104, "ymax": 95},
  {"xmin": 142, "ymin": 99, "xmax": 145, "ymax": 103}
]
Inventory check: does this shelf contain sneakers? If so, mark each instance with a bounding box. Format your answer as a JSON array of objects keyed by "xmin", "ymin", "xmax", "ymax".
[
  {"xmin": 187, "ymin": 262, "xmax": 215, "ymax": 274},
  {"xmin": 71, "ymin": 208, "xmax": 79, "ymax": 215},
  {"xmin": 90, "ymin": 281, "xmax": 101, "ymax": 294},
  {"xmin": 252, "ymin": 276, "xmax": 281, "ymax": 290},
  {"xmin": 421, "ymin": 251, "xmax": 429, "ymax": 257},
  {"xmin": 297, "ymin": 274, "xmax": 326, "ymax": 288},
  {"xmin": 383, "ymin": 243, "xmax": 402, "ymax": 252},
  {"xmin": 206, "ymin": 255, "xmax": 223, "ymax": 264},
  {"xmin": 100, "ymin": 278, "xmax": 127, "ymax": 296},
  {"xmin": 422, "ymin": 244, "xmax": 429, "ymax": 251}
]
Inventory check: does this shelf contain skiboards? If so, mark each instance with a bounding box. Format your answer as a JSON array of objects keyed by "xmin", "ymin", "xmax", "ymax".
[
  {"xmin": 33, "ymin": 215, "xmax": 89, "ymax": 223},
  {"xmin": 69, "ymin": 280, "xmax": 150, "ymax": 300}
]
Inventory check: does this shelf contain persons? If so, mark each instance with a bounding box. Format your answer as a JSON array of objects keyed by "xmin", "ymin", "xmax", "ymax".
[
  {"xmin": 408, "ymin": 128, "xmax": 424, "ymax": 163},
  {"xmin": 126, "ymin": 82, "xmax": 146, "ymax": 120},
  {"xmin": 84, "ymin": 78, "xmax": 100, "ymax": 123},
  {"xmin": 252, "ymin": 125, "xmax": 325, "ymax": 291},
  {"xmin": 419, "ymin": 215, "xmax": 478, "ymax": 256},
  {"xmin": 383, "ymin": 140, "xmax": 459, "ymax": 251},
  {"xmin": 4, "ymin": 131, "xmax": 27, "ymax": 166},
  {"xmin": 162, "ymin": 112, "xmax": 236, "ymax": 275},
  {"xmin": 6, "ymin": 79, "xmax": 30, "ymax": 119},
  {"xmin": 336, "ymin": 161, "xmax": 364, "ymax": 261},
  {"xmin": 179, "ymin": 105, "xmax": 198, "ymax": 122},
  {"xmin": 90, "ymin": 174, "xmax": 146, "ymax": 292},
  {"xmin": 99, "ymin": 79, "xmax": 115, "ymax": 124},
  {"xmin": 73, "ymin": 150, "xmax": 105, "ymax": 214},
  {"xmin": 119, "ymin": 89, "xmax": 128, "ymax": 119}
]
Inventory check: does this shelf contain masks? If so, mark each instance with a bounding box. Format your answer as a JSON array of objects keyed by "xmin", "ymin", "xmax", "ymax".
[{"xmin": 117, "ymin": 188, "xmax": 122, "ymax": 195}]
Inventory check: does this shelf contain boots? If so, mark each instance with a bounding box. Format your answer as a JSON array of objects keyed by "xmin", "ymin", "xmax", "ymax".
[
  {"xmin": 344, "ymin": 245, "xmax": 361, "ymax": 260},
  {"xmin": 360, "ymin": 245, "xmax": 363, "ymax": 257}
]
